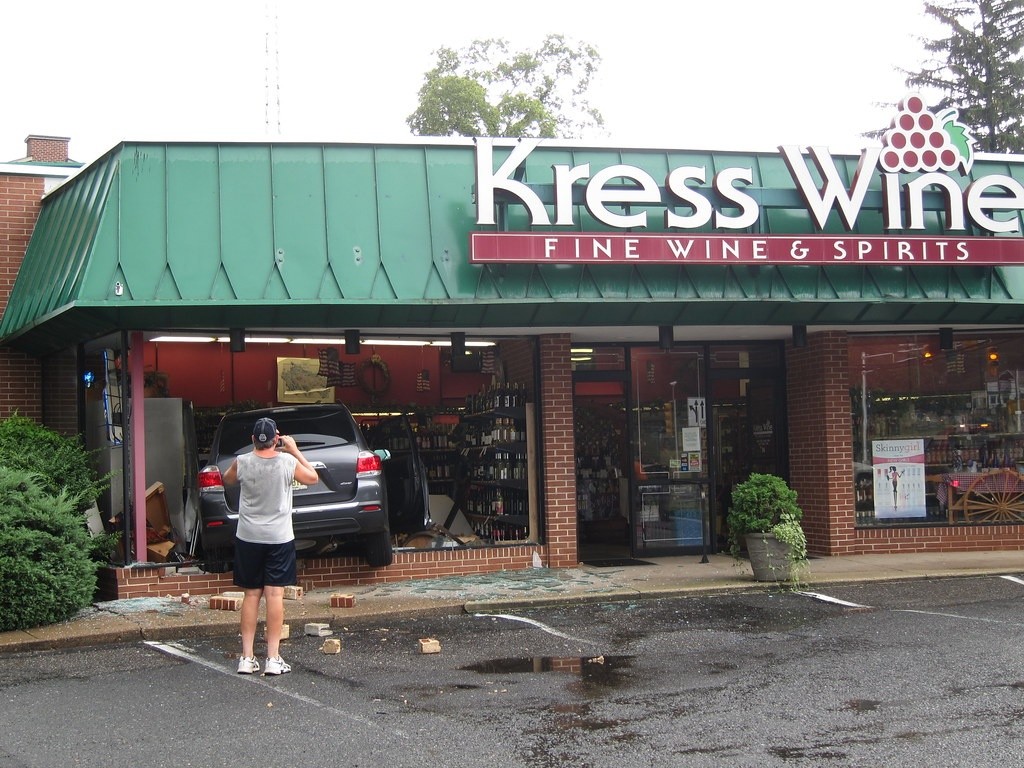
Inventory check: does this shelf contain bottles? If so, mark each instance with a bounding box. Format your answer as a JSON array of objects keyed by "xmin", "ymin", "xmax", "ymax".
[
  {"xmin": 384, "ymin": 375, "xmax": 529, "ymax": 541},
  {"xmin": 851, "ymin": 384, "xmax": 1024, "ymax": 524}
]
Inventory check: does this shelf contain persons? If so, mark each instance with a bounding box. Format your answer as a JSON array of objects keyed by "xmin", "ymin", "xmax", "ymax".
[{"xmin": 223, "ymin": 418, "xmax": 319, "ymax": 676}]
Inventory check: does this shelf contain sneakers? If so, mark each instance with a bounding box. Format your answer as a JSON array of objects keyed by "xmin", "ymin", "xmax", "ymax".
[
  {"xmin": 264, "ymin": 654, "xmax": 292, "ymax": 675},
  {"xmin": 237, "ymin": 655, "xmax": 260, "ymax": 674}
]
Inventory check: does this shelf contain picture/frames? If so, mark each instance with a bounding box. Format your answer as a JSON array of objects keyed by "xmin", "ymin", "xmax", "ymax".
[{"xmin": 277, "ymin": 356, "xmax": 335, "ymax": 404}]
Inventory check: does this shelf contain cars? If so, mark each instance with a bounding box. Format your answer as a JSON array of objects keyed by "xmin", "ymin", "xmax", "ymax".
[
  {"xmin": 854, "ymin": 460, "xmax": 875, "ymax": 489},
  {"xmin": 196, "ymin": 401, "xmax": 430, "ymax": 576}
]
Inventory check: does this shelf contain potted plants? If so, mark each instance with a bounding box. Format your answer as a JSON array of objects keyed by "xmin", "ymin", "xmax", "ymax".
[{"xmin": 726, "ymin": 472, "xmax": 818, "ymax": 594}]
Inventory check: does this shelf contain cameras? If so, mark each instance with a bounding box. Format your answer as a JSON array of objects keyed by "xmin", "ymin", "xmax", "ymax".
[{"xmin": 275, "ymin": 439, "xmax": 283, "ymax": 447}]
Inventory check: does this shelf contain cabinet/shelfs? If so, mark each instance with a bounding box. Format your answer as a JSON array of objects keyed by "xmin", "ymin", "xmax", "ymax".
[
  {"xmin": 857, "ymin": 431, "xmax": 1024, "ymax": 496},
  {"xmin": 459, "ymin": 401, "xmax": 539, "ymax": 545},
  {"xmin": 389, "ymin": 449, "xmax": 455, "ymax": 483}
]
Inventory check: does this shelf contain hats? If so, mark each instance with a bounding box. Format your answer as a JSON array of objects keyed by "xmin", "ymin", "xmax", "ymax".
[{"xmin": 253, "ymin": 417, "xmax": 280, "ymax": 443}]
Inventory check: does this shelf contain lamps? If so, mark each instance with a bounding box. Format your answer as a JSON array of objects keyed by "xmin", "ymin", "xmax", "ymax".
[
  {"xmin": 792, "ymin": 325, "xmax": 808, "ymax": 348},
  {"xmin": 450, "ymin": 332, "xmax": 465, "ymax": 358},
  {"xmin": 344, "ymin": 330, "xmax": 360, "ymax": 355},
  {"xmin": 939, "ymin": 328, "xmax": 954, "ymax": 352},
  {"xmin": 659, "ymin": 326, "xmax": 674, "ymax": 350},
  {"xmin": 229, "ymin": 328, "xmax": 246, "ymax": 353}
]
